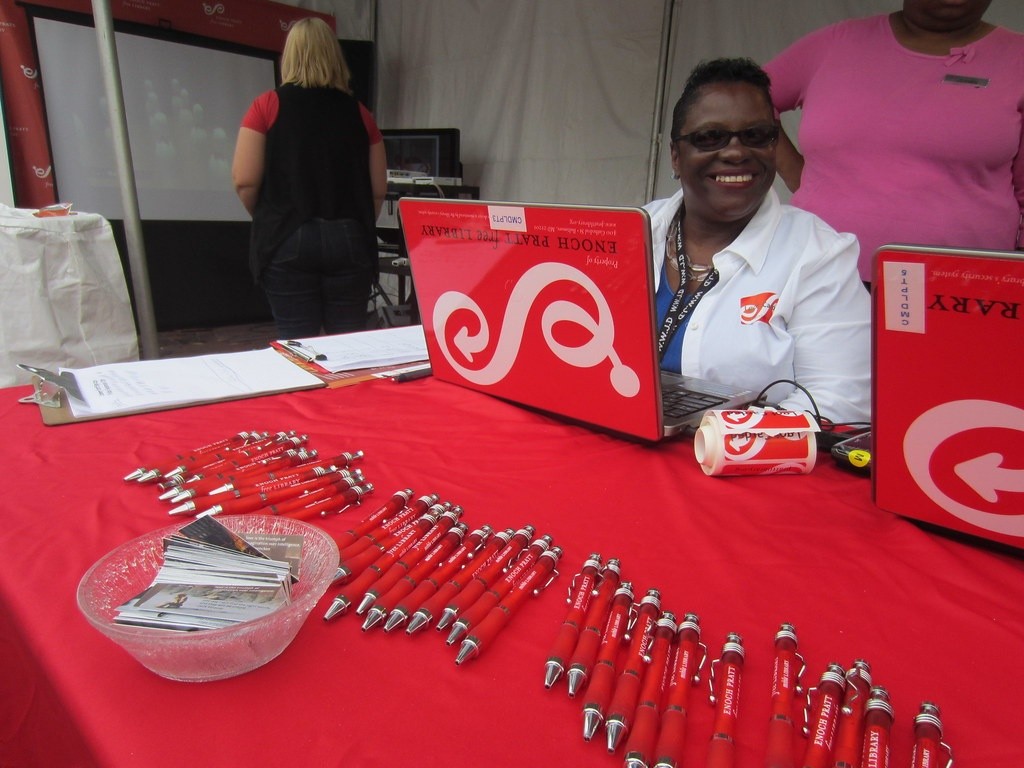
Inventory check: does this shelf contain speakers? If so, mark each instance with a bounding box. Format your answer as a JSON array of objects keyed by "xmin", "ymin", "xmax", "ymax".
[{"xmin": 339, "ymin": 39, "xmax": 377, "ymax": 110}]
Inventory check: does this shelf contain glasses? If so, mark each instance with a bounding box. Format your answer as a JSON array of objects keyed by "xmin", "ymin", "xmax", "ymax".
[{"xmin": 672, "ymin": 118, "xmax": 782, "ymax": 152}]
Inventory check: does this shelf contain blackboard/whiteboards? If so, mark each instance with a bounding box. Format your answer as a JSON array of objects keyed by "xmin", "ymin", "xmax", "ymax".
[{"xmin": 13, "ymin": 1, "xmax": 280, "ymax": 224}]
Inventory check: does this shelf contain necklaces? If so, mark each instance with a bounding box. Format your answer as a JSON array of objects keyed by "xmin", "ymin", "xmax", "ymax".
[{"xmin": 664, "ymin": 214, "xmax": 713, "ymax": 284}]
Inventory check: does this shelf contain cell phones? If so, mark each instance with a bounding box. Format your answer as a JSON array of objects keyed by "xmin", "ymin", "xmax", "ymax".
[{"xmin": 832, "ymin": 429, "xmax": 872, "ymax": 476}]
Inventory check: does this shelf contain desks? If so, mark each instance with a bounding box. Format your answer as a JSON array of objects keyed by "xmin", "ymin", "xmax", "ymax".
[
  {"xmin": 0, "ymin": 202, "xmax": 140, "ymax": 390},
  {"xmin": 0, "ymin": 359, "xmax": 1024, "ymax": 768}
]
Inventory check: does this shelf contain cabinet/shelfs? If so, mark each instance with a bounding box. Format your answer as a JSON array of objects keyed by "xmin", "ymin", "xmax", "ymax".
[{"xmin": 368, "ymin": 183, "xmax": 481, "ymax": 330}]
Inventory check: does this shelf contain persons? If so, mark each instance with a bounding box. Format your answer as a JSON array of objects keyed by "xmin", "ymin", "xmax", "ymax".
[
  {"xmin": 231, "ymin": 17, "xmax": 389, "ymax": 342},
  {"xmin": 639, "ymin": 59, "xmax": 874, "ymax": 431},
  {"xmin": 759, "ymin": 0, "xmax": 1024, "ymax": 296}
]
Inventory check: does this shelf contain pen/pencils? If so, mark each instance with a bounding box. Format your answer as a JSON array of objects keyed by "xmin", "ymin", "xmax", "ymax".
[
  {"xmin": 909, "ymin": 700, "xmax": 954, "ymax": 768},
  {"xmin": 799, "ymin": 662, "xmax": 847, "ymax": 768},
  {"xmin": 396, "ymin": 367, "xmax": 432, "ymax": 383},
  {"xmin": 654, "ymin": 611, "xmax": 709, "ymax": 768},
  {"xmin": 625, "ymin": 610, "xmax": 677, "ymax": 768},
  {"xmin": 322, "ymin": 486, "xmax": 563, "ymax": 665},
  {"xmin": 568, "ymin": 558, "xmax": 622, "ymax": 695},
  {"xmin": 606, "ymin": 587, "xmax": 662, "ymax": 753},
  {"xmin": 122, "ymin": 430, "xmax": 373, "ymax": 523},
  {"xmin": 708, "ymin": 632, "xmax": 746, "ymax": 768},
  {"xmin": 833, "ymin": 657, "xmax": 871, "ymax": 768},
  {"xmin": 766, "ymin": 622, "xmax": 807, "ymax": 768},
  {"xmin": 861, "ymin": 684, "xmax": 894, "ymax": 768},
  {"xmin": 543, "ymin": 553, "xmax": 602, "ymax": 688},
  {"xmin": 584, "ymin": 582, "xmax": 635, "ymax": 741}
]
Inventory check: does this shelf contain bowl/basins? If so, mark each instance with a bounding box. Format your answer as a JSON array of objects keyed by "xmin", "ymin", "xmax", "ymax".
[{"xmin": 76, "ymin": 514, "xmax": 341, "ymax": 683}]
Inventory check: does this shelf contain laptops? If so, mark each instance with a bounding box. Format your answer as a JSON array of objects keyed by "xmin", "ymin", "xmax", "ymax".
[
  {"xmin": 397, "ymin": 196, "xmax": 768, "ymax": 441},
  {"xmin": 869, "ymin": 245, "xmax": 1024, "ymax": 558}
]
